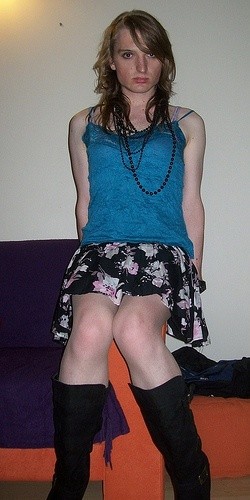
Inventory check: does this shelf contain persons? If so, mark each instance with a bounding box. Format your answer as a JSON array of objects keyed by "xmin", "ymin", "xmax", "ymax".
[{"xmin": 47, "ymin": 11, "xmax": 212, "ymax": 499}]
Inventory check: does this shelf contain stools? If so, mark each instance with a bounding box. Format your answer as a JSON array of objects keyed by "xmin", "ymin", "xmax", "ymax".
[{"xmin": 188, "ymin": 394, "xmax": 250, "ymax": 480}]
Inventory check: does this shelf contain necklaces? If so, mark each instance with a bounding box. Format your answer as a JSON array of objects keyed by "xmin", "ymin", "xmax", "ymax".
[{"xmin": 113, "ymin": 110, "xmax": 176, "ymax": 196}]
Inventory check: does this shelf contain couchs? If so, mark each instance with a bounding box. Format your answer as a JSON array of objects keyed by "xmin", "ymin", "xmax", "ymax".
[{"xmin": 0, "ymin": 238, "xmax": 166, "ymax": 500}]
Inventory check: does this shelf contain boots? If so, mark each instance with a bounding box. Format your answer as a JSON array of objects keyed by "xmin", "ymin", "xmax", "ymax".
[
  {"xmin": 127, "ymin": 376, "xmax": 211, "ymax": 500},
  {"xmin": 47, "ymin": 373, "xmax": 108, "ymax": 500}
]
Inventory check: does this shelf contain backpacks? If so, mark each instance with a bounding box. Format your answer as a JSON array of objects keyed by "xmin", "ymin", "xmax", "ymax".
[{"xmin": 170, "ymin": 346, "xmax": 250, "ymax": 398}]
